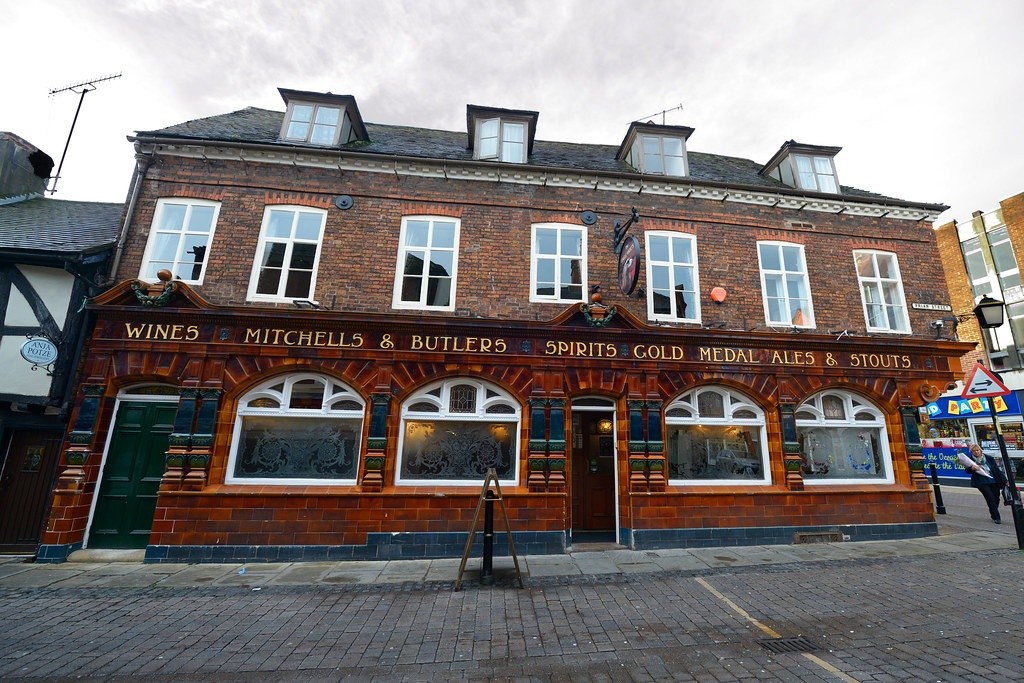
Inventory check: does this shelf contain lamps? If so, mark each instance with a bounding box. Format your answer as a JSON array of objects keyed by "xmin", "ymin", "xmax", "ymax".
[
  {"xmin": 598, "ymin": 419, "xmax": 613, "ymax": 434},
  {"xmin": 954, "ymin": 294, "xmax": 1004, "ymax": 329}
]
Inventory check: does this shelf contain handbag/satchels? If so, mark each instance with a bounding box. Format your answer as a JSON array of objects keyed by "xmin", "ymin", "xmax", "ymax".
[{"xmin": 1002, "ymin": 485, "xmax": 1013, "ymax": 506}]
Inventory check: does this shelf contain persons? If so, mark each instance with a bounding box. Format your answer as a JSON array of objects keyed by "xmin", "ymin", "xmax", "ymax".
[{"xmin": 965, "ymin": 444, "xmax": 1008, "ymax": 524}]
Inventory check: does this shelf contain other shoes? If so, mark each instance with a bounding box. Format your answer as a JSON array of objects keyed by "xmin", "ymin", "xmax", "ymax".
[{"xmin": 994, "ymin": 519, "xmax": 1001, "ymax": 524}]
